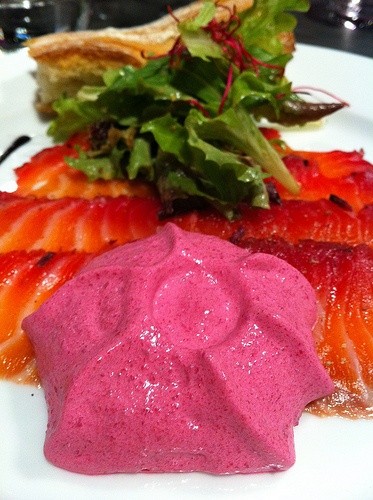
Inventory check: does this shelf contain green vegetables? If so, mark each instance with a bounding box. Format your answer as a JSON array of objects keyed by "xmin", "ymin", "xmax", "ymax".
[{"xmin": 53, "ymin": 4, "xmax": 350, "ymax": 225}]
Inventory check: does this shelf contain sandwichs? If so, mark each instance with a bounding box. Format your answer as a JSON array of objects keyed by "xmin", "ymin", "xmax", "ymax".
[{"xmin": 31, "ymin": 1, "xmax": 251, "ymax": 118}]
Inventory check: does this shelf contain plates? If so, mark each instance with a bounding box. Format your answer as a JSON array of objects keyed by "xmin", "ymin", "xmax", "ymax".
[{"xmin": 0, "ymin": 39, "xmax": 373, "ymax": 476}]
